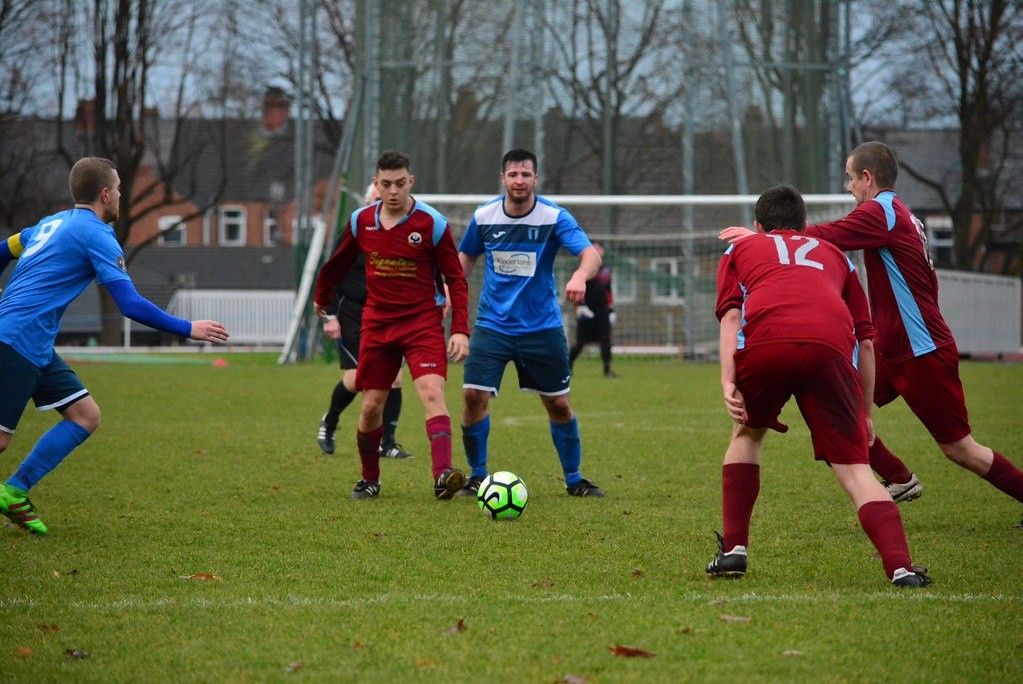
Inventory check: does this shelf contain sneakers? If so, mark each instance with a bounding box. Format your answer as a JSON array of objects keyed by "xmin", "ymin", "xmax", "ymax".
[
  {"xmin": 464, "ymin": 475, "xmax": 486, "ymax": 495},
  {"xmin": 880, "ymin": 473, "xmax": 923, "ymax": 503},
  {"xmin": 351, "ymin": 480, "xmax": 382, "ymax": 498},
  {"xmin": 565, "ymin": 478, "xmax": 604, "ymax": 497},
  {"xmin": 0, "ymin": 482, "xmax": 48, "ymax": 535},
  {"xmin": 892, "ymin": 565, "xmax": 932, "ymax": 588},
  {"xmin": 704, "ymin": 530, "xmax": 747, "ymax": 576},
  {"xmin": 317, "ymin": 413, "xmax": 337, "ymax": 454},
  {"xmin": 378, "ymin": 442, "xmax": 415, "ymax": 459},
  {"xmin": 434, "ymin": 468, "xmax": 466, "ymax": 500}
]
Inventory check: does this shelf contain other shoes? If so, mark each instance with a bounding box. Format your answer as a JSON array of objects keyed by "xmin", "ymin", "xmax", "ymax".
[{"xmin": 605, "ymin": 369, "xmax": 615, "ymax": 377}]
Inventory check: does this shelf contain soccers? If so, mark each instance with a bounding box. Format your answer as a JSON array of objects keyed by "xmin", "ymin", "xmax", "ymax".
[{"xmin": 477, "ymin": 470, "xmax": 528, "ymax": 520}]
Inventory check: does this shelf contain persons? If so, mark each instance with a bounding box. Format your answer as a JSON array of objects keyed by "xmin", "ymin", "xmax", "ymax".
[
  {"xmin": 313, "ymin": 150, "xmax": 471, "ymax": 500},
  {"xmin": 0, "ymin": 155, "xmax": 229, "ymax": 535},
  {"xmin": 717, "ymin": 141, "xmax": 1023, "ymax": 528},
  {"xmin": 568, "ymin": 240, "xmax": 617, "ymax": 378},
  {"xmin": 706, "ymin": 183, "xmax": 933, "ymax": 585},
  {"xmin": 443, "ymin": 149, "xmax": 605, "ymax": 498}
]
